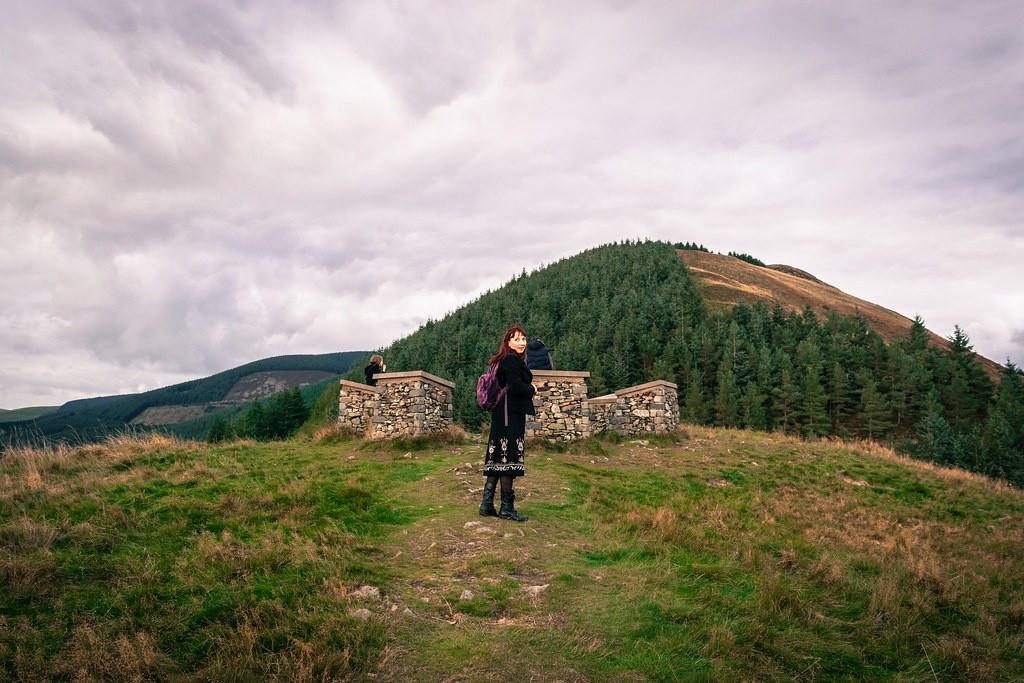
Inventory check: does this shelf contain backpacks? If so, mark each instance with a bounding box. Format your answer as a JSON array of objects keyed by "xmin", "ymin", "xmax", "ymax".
[{"xmin": 476, "ymin": 358, "xmax": 509, "ymax": 413}]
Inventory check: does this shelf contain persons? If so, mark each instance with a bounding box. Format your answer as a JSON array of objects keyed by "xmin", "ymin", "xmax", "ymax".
[
  {"xmin": 478, "ymin": 326, "xmax": 538, "ymax": 522},
  {"xmin": 365, "ymin": 355, "xmax": 387, "ymax": 386},
  {"xmin": 525, "ymin": 335, "xmax": 554, "ymax": 370}
]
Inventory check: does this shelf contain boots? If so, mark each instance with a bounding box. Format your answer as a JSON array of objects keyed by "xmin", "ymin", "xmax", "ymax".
[
  {"xmin": 499, "ymin": 489, "xmax": 529, "ymax": 522},
  {"xmin": 479, "ymin": 482, "xmax": 499, "ymax": 517}
]
